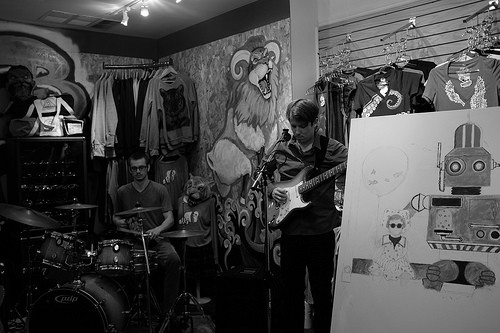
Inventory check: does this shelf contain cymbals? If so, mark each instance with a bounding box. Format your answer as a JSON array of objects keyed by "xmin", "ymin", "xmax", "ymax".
[
  {"xmin": 54, "ymin": 202, "xmax": 97, "ymax": 209},
  {"xmin": 114, "ymin": 206, "xmax": 164, "ymax": 215},
  {"xmin": 0, "ymin": 202, "xmax": 58, "ymax": 226},
  {"xmin": 160, "ymin": 229, "xmax": 205, "ymax": 237}
]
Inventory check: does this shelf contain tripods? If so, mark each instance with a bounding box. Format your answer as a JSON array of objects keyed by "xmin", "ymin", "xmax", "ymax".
[{"xmin": 158, "ymin": 238, "xmax": 216, "ymax": 333}]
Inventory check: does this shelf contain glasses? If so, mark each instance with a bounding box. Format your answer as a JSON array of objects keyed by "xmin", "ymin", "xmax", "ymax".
[{"xmin": 130, "ymin": 165, "xmax": 146, "ymax": 172}]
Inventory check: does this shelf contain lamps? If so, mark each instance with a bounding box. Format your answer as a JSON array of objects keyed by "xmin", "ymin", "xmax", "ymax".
[
  {"xmin": 120, "ymin": 6, "xmax": 131, "ymax": 26},
  {"xmin": 140, "ymin": 3, "xmax": 150, "ymax": 18}
]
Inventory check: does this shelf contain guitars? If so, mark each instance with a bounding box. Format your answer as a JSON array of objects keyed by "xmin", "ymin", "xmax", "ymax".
[{"xmin": 261, "ymin": 159, "xmax": 346, "ymax": 229}]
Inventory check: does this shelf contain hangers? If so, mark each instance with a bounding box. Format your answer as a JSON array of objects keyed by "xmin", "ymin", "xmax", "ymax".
[{"xmin": 92, "ymin": 7, "xmax": 500, "ymax": 167}]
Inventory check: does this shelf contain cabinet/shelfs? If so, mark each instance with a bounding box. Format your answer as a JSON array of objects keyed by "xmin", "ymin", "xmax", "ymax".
[{"xmin": 6, "ymin": 137, "xmax": 88, "ymax": 223}]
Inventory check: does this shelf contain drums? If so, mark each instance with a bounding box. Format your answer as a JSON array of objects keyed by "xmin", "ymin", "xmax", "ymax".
[
  {"xmin": 34, "ymin": 230, "xmax": 86, "ymax": 272},
  {"xmin": 25, "ymin": 272, "xmax": 131, "ymax": 333},
  {"xmin": 131, "ymin": 249, "xmax": 158, "ymax": 272},
  {"xmin": 94, "ymin": 239, "xmax": 135, "ymax": 276}
]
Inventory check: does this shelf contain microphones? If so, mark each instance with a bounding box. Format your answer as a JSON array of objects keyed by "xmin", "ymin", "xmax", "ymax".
[{"xmin": 282, "ymin": 133, "xmax": 291, "ymax": 142}]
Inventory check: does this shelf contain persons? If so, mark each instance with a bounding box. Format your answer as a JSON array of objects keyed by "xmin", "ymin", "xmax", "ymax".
[
  {"xmin": 254, "ymin": 98, "xmax": 349, "ymax": 333},
  {"xmin": 112, "ymin": 153, "xmax": 180, "ymax": 307}
]
